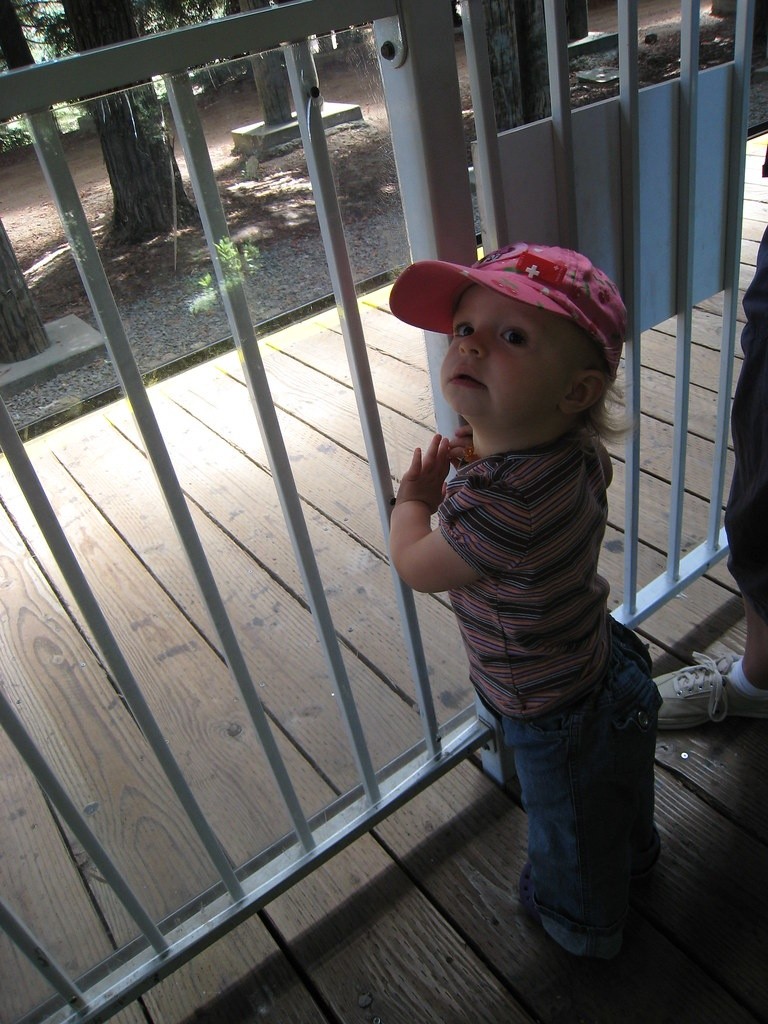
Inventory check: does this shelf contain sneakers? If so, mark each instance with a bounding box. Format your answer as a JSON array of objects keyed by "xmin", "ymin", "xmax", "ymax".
[{"xmin": 653, "ymin": 651, "xmax": 768, "ymax": 731}]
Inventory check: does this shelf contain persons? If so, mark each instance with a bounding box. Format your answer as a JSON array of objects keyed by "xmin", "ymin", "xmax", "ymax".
[
  {"xmin": 652, "ymin": 146, "xmax": 767, "ymax": 728},
  {"xmin": 390, "ymin": 245, "xmax": 662, "ymax": 959}
]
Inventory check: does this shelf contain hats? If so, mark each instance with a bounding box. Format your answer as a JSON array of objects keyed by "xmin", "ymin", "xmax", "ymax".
[{"xmin": 389, "ymin": 241, "xmax": 627, "ymax": 380}]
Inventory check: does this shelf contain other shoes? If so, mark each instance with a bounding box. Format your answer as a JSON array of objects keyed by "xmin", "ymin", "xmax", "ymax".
[
  {"xmin": 519, "ymin": 860, "xmax": 540, "ymax": 924},
  {"xmin": 631, "ymin": 857, "xmax": 656, "ymax": 880}
]
